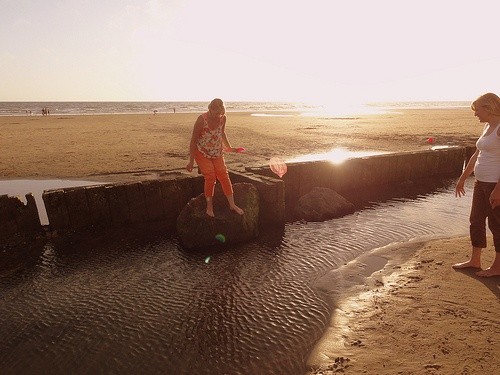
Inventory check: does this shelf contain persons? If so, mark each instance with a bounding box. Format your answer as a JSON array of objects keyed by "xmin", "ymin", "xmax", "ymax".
[
  {"xmin": 186, "ymin": 98, "xmax": 244, "ymax": 217},
  {"xmin": 452, "ymin": 93, "xmax": 500, "ymax": 277}
]
